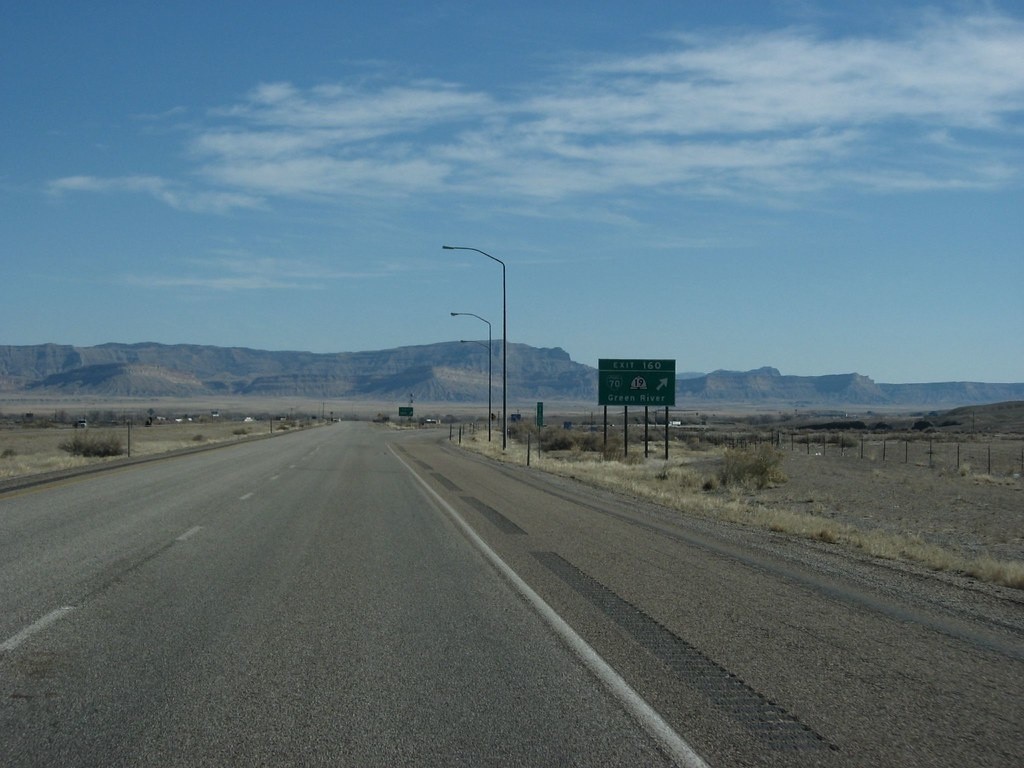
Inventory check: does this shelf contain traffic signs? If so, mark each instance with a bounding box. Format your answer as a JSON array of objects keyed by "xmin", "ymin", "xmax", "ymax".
[{"xmin": 598, "ymin": 359, "xmax": 675, "ymax": 406}]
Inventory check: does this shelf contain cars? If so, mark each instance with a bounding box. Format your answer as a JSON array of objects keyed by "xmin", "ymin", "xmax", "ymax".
[{"xmin": 72, "ymin": 419, "xmax": 87, "ymax": 428}]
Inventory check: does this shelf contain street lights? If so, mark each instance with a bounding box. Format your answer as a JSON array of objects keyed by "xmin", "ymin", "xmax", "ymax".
[
  {"xmin": 450, "ymin": 312, "xmax": 491, "ymax": 442},
  {"xmin": 443, "ymin": 245, "xmax": 507, "ymax": 450},
  {"xmin": 460, "ymin": 340, "xmax": 491, "ymax": 442}
]
